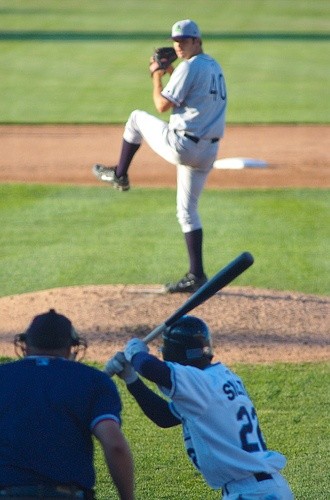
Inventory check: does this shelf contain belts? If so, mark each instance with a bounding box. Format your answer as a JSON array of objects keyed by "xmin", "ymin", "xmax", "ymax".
[
  {"xmin": 174, "ymin": 130, "xmax": 219, "ymax": 144},
  {"xmin": 221, "ymin": 472, "xmax": 273, "ymax": 496}
]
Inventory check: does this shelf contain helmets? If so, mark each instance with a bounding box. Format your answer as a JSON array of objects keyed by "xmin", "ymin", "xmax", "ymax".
[{"xmin": 161, "ymin": 315, "xmax": 215, "ymax": 364}]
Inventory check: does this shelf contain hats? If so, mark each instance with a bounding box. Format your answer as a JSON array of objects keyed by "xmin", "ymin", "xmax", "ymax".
[
  {"xmin": 25, "ymin": 309, "xmax": 77, "ymax": 350},
  {"xmin": 170, "ymin": 19, "xmax": 201, "ymax": 41}
]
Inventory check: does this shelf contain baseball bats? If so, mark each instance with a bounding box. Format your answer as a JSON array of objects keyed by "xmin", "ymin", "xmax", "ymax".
[{"xmin": 144, "ymin": 251, "xmax": 254, "ymax": 342}]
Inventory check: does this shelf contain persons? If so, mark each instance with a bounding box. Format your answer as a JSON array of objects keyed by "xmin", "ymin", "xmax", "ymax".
[
  {"xmin": 0, "ymin": 309, "xmax": 135, "ymax": 500},
  {"xmin": 93, "ymin": 19, "xmax": 225, "ymax": 291},
  {"xmin": 105, "ymin": 316, "xmax": 294, "ymax": 500}
]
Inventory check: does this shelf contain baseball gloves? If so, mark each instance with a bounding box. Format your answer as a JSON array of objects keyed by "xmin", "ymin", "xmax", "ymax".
[{"xmin": 148, "ymin": 45, "xmax": 178, "ymax": 74}]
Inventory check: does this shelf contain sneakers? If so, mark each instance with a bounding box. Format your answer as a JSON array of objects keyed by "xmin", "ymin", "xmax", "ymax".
[
  {"xmin": 166, "ymin": 271, "xmax": 208, "ymax": 294},
  {"xmin": 92, "ymin": 162, "xmax": 130, "ymax": 191}
]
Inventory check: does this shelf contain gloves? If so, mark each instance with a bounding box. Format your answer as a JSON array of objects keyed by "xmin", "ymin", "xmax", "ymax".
[
  {"xmin": 106, "ymin": 351, "xmax": 138, "ymax": 385},
  {"xmin": 124, "ymin": 337, "xmax": 150, "ymax": 362}
]
